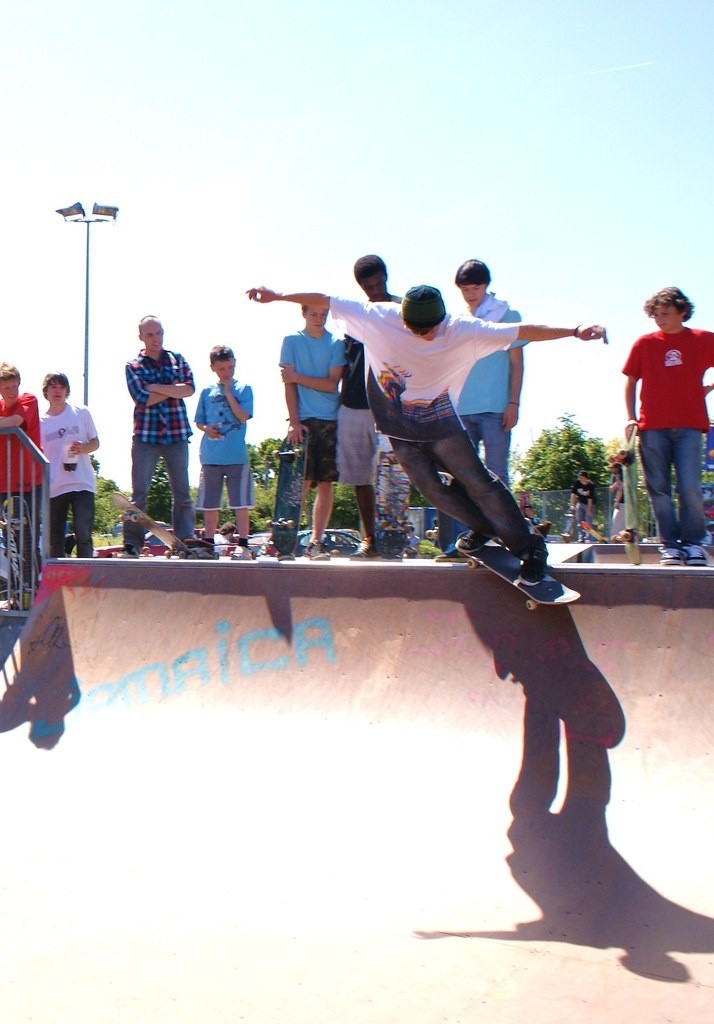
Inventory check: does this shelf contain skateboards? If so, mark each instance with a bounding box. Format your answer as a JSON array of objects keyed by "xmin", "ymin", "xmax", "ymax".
[
  {"xmin": 579, "ymin": 520, "xmax": 607, "ymax": 543},
  {"xmin": 454, "ymin": 535, "xmax": 582, "ymax": 610},
  {"xmin": 606, "ymin": 425, "xmax": 642, "ymax": 566},
  {"xmin": 373, "ymin": 447, "xmax": 411, "ymax": 559},
  {"xmin": 1, "ymin": 496, "xmax": 40, "ymax": 591},
  {"xmin": 183, "ymin": 537, "xmax": 265, "ymax": 561},
  {"xmin": 110, "ymin": 492, "xmax": 195, "ymax": 560},
  {"xmin": 265, "ymin": 428, "xmax": 310, "ymax": 556}
]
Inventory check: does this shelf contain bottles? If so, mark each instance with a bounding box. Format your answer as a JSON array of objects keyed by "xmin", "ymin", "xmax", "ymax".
[{"xmin": 62, "ymin": 427, "xmax": 79, "ymax": 471}]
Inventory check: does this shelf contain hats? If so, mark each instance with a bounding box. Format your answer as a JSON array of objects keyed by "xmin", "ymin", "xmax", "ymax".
[{"xmin": 401, "ymin": 285, "xmax": 446, "ymax": 327}]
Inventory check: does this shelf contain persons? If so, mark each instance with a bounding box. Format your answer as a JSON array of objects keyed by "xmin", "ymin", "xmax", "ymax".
[
  {"xmin": 0, "ymin": 255, "xmax": 627, "ymax": 615},
  {"xmin": 622, "ymin": 288, "xmax": 714, "ymax": 570}
]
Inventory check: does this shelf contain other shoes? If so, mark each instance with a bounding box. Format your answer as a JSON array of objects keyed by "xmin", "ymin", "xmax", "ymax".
[
  {"xmin": 187, "ymin": 550, "xmax": 212, "ymax": 558},
  {"xmin": 231, "ymin": 546, "xmax": 252, "ymax": 560}
]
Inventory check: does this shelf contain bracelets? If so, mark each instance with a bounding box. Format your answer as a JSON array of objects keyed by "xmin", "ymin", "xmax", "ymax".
[{"xmin": 573, "ymin": 324, "xmax": 582, "ymax": 338}]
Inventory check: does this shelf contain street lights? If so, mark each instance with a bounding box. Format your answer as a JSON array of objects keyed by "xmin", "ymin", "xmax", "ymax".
[{"xmin": 55, "ymin": 201, "xmax": 120, "ymax": 404}]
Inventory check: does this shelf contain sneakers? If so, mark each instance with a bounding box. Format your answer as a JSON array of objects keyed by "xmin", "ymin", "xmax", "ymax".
[
  {"xmin": 656, "ymin": 546, "xmax": 682, "ymax": 565},
  {"xmin": 436, "ymin": 551, "xmax": 474, "ymax": 562},
  {"xmin": 350, "ymin": 540, "xmax": 382, "ymax": 561},
  {"xmin": 117, "ymin": 544, "xmax": 139, "ymax": 558},
  {"xmin": 455, "ymin": 528, "xmax": 491, "ymax": 552},
  {"xmin": 304, "ymin": 542, "xmax": 331, "ymax": 561},
  {"xmin": 518, "ymin": 548, "xmax": 548, "ymax": 586},
  {"xmin": 680, "ymin": 542, "xmax": 708, "ymax": 565},
  {"xmin": 277, "ymin": 552, "xmax": 295, "ymax": 561}
]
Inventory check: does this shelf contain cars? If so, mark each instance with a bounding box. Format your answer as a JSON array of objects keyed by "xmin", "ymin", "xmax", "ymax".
[{"xmin": 247, "ymin": 528, "xmax": 387, "ymax": 557}]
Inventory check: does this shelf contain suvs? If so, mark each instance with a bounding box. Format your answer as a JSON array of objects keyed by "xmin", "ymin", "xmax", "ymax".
[{"xmin": 92, "ymin": 521, "xmax": 238, "ymax": 558}]
